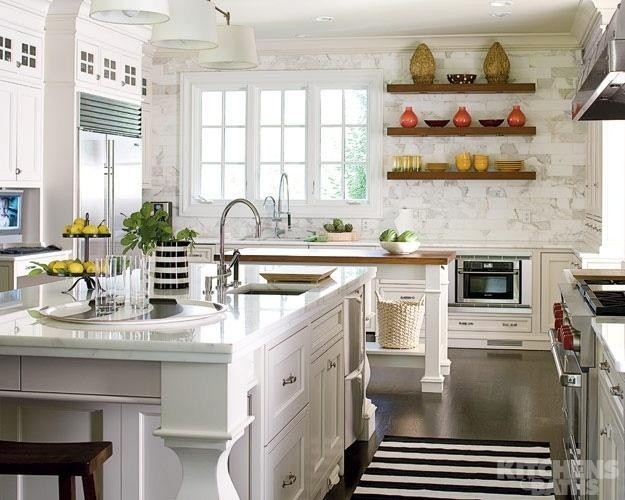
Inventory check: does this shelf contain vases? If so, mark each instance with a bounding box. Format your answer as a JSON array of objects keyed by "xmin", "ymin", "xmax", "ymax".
[
  {"xmin": 399, "ymin": 105, "xmax": 419, "ymax": 129},
  {"xmin": 453, "ymin": 105, "xmax": 472, "ymax": 128},
  {"xmin": 504, "ymin": 104, "xmax": 528, "ymax": 128}
]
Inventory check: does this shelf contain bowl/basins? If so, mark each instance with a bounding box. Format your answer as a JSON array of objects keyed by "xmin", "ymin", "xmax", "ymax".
[
  {"xmin": 478, "ymin": 119, "xmax": 506, "ymax": 127},
  {"xmin": 447, "ymin": 74, "xmax": 477, "ymax": 85},
  {"xmin": 455, "ymin": 151, "xmax": 490, "ymax": 173},
  {"xmin": 423, "ymin": 119, "xmax": 451, "ymax": 128},
  {"xmin": 379, "ymin": 242, "xmax": 421, "ymax": 255}
]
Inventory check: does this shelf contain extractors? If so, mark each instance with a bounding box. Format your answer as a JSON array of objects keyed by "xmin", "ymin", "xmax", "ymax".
[{"xmin": 571, "ymin": 38, "xmax": 625, "ymax": 122}]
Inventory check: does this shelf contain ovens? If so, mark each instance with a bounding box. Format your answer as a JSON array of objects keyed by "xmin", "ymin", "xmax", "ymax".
[{"xmin": 546, "ymin": 328, "xmax": 598, "ymax": 500}]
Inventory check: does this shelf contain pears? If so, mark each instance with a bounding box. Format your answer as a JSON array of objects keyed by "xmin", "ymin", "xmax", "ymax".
[
  {"xmin": 48, "ymin": 260, "xmax": 109, "ymax": 274},
  {"xmin": 63, "ymin": 218, "xmax": 109, "ymax": 235}
]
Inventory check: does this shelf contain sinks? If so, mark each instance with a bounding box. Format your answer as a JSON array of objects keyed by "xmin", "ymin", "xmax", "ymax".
[
  {"xmin": 237, "ymin": 237, "xmax": 304, "ymax": 242},
  {"xmin": 226, "ymin": 281, "xmax": 317, "ymax": 296}
]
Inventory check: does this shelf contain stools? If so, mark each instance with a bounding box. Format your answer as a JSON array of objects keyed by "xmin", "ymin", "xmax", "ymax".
[{"xmin": 0, "ymin": 438, "xmax": 114, "ymax": 500}]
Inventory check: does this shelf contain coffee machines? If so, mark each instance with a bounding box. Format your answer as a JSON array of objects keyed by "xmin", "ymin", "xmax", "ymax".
[{"xmin": 149, "ymin": 200, "xmax": 173, "ymax": 228}]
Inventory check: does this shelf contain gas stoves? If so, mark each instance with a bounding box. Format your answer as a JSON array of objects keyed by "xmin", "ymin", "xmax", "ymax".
[{"xmin": 577, "ymin": 278, "xmax": 625, "ymax": 315}]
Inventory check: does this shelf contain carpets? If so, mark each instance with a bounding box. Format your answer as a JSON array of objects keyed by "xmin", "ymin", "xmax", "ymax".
[{"xmin": 351, "ymin": 433, "xmax": 556, "ymax": 500}]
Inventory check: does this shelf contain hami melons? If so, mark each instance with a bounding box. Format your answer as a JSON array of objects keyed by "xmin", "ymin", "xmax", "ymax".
[
  {"xmin": 397, "ymin": 231, "xmax": 417, "ymax": 241},
  {"xmin": 379, "ymin": 229, "xmax": 396, "ymax": 242}
]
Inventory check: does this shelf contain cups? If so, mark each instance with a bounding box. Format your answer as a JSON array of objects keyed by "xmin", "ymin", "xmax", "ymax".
[
  {"xmin": 93, "ymin": 255, "xmax": 149, "ymax": 317},
  {"xmin": 391, "ymin": 155, "xmax": 422, "ymax": 173},
  {"xmin": 302, "ymin": 234, "xmax": 328, "ymax": 243}
]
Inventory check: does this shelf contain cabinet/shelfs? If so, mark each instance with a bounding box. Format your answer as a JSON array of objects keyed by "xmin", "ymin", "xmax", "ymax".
[
  {"xmin": 308, "ymin": 297, "xmax": 349, "ymax": 500},
  {"xmin": 374, "ymin": 281, "xmax": 429, "ymax": 340},
  {"xmin": 0, "ymin": 357, "xmax": 254, "ymax": 499},
  {"xmin": 534, "ymin": 245, "xmax": 574, "ymax": 351},
  {"xmin": 595, "ymin": 322, "xmax": 625, "ymax": 500},
  {"xmin": 0, "ymin": 23, "xmax": 46, "ymax": 73},
  {"xmin": 74, "ymin": 34, "xmax": 145, "ymax": 97},
  {"xmin": 249, "ymin": 321, "xmax": 306, "ymax": 500},
  {"xmin": 583, "ymin": 113, "xmax": 625, "ymax": 252},
  {"xmin": 449, "ymin": 305, "xmax": 533, "ymax": 351},
  {"xmin": 383, "ymin": 80, "xmax": 540, "ymax": 184},
  {"xmin": 140, "ymin": 98, "xmax": 152, "ymax": 192},
  {"xmin": 2, "ymin": 72, "xmax": 46, "ymax": 189},
  {"xmin": 0, "ymin": 248, "xmax": 75, "ymax": 292}
]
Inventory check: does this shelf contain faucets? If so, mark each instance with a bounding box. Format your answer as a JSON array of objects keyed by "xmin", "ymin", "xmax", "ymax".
[
  {"xmin": 263, "ymin": 196, "xmax": 276, "ymax": 218},
  {"xmin": 214, "ymin": 198, "xmax": 262, "ymax": 289},
  {"xmin": 277, "ymin": 173, "xmax": 293, "ymax": 232}
]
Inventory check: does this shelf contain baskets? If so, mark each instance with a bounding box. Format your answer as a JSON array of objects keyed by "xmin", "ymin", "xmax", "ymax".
[{"xmin": 377, "ymin": 298, "xmax": 425, "ymax": 349}]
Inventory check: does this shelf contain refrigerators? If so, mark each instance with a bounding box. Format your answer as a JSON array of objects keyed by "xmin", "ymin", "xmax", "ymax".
[{"xmin": 76, "ymin": 129, "xmax": 144, "ymax": 261}]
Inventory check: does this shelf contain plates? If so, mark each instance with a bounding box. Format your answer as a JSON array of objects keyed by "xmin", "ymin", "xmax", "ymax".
[
  {"xmin": 494, "ymin": 160, "xmax": 525, "ymax": 173},
  {"xmin": 425, "ymin": 162, "xmax": 449, "ymax": 173}
]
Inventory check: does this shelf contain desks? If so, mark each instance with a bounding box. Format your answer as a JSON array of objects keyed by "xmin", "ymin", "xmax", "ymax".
[{"xmin": 212, "ymin": 248, "xmax": 455, "ymax": 396}]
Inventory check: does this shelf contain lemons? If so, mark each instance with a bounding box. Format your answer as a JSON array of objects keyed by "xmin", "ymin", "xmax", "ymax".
[{"xmin": 324, "ymin": 219, "xmax": 352, "ymax": 232}]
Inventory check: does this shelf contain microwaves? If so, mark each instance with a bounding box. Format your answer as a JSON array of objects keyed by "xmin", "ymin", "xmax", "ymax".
[{"xmin": 455, "ymin": 257, "xmax": 521, "ymax": 304}]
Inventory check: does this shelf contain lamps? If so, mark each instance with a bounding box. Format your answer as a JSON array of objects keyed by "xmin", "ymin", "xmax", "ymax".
[{"xmin": 86, "ymin": 0, "xmax": 260, "ymax": 72}]
